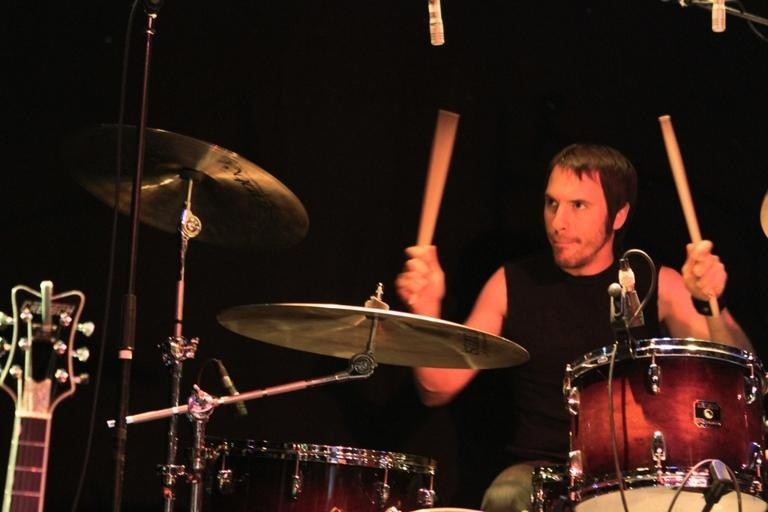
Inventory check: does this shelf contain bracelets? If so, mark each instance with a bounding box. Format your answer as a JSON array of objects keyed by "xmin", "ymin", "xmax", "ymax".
[{"xmin": 693, "ymin": 293, "xmax": 727, "ymax": 316}]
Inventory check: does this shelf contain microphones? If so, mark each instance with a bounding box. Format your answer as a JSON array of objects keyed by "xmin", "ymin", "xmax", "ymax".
[
  {"xmin": 618, "ymin": 258, "xmax": 645, "ymax": 328},
  {"xmin": 429, "ymin": 0, "xmax": 445, "ymax": 46},
  {"xmin": 711, "ymin": 0, "xmax": 726, "ymax": 32}
]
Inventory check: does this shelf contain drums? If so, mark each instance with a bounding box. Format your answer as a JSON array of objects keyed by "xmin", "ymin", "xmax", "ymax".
[
  {"xmin": 563, "ymin": 337, "xmax": 768, "ymax": 511},
  {"xmin": 204, "ymin": 438, "xmax": 437, "ymax": 512}
]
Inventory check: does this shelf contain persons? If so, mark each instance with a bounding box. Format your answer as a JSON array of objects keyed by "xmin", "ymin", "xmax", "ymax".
[{"xmin": 396, "ymin": 144, "xmax": 756, "ymax": 510}]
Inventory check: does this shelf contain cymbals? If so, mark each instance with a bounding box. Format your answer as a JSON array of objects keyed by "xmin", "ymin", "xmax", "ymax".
[
  {"xmin": 217, "ymin": 303, "xmax": 530, "ymax": 369},
  {"xmin": 56, "ymin": 123, "xmax": 310, "ymax": 251}
]
